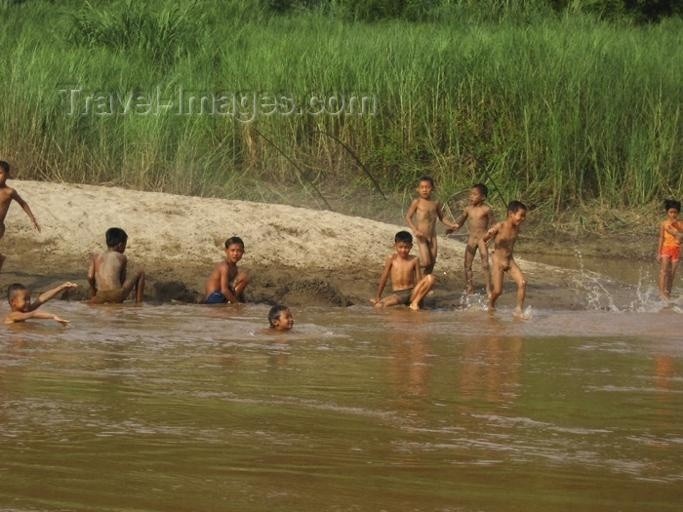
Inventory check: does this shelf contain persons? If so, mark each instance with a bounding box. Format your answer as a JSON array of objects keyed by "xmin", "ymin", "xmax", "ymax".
[
  {"xmin": 203, "ymin": 236, "xmax": 250, "ymax": 304},
  {"xmin": 255, "ymin": 306, "xmax": 295, "ymax": 335},
  {"xmin": 84, "ymin": 227, "xmax": 146, "ymax": 303},
  {"xmin": 373, "ymin": 231, "xmax": 435, "ymax": 311},
  {"xmin": 445, "ymin": 184, "xmax": 497, "ymax": 298},
  {"xmin": 476, "ymin": 199, "xmax": 528, "ymax": 316},
  {"xmin": 1, "ymin": 160, "xmax": 42, "ymax": 273},
  {"xmin": 654, "ymin": 198, "xmax": 683, "ymax": 297},
  {"xmin": 405, "ymin": 176, "xmax": 459, "ymax": 275},
  {"xmin": 2, "ymin": 281, "xmax": 79, "ymax": 327}
]
